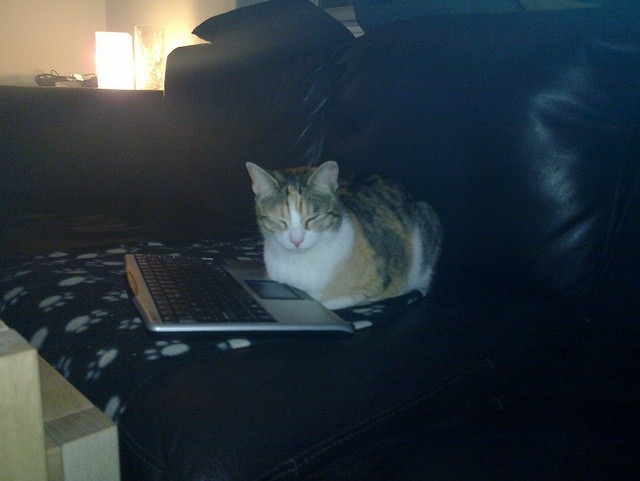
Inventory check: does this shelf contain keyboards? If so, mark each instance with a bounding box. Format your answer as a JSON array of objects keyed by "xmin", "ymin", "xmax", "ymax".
[{"xmin": 124, "ymin": 251, "xmax": 355, "ymax": 340}]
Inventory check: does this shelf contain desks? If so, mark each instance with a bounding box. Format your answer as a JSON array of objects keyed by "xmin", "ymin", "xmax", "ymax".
[{"xmin": 0, "ymin": 317, "xmax": 123, "ymax": 477}]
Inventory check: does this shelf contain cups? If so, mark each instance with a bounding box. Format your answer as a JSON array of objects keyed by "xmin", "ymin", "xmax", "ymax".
[{"xmin": 133, "ymin": 24, "xmax": 166, "ymax": 92}]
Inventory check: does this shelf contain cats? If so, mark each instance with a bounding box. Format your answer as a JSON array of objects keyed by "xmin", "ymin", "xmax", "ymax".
[{"xmin": 245, "ymin": 161, "xmax": 445, "ymax": 312}]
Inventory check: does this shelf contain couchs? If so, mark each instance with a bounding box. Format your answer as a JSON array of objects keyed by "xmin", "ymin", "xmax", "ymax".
[{"xmin": 0, "ymin": 25, "xmax": 638, "ymax": 473}]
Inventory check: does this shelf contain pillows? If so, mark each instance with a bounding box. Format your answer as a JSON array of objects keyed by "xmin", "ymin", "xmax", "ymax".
[
  {"xmin": 349, "ymin": 0, "xmax": 523, "ymax": 42},
  {"xmin": 189, "ymin": 7, "xmax": 349, "ymax": 53}
]
